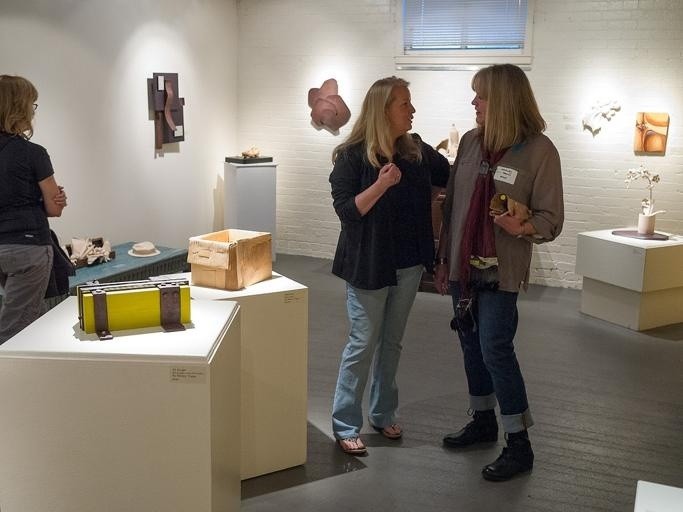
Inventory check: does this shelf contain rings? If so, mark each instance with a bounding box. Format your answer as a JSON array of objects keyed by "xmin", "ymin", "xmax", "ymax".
[{"xmin": 397, "ymin": 174, "xmax": 400, "ymax": 177}]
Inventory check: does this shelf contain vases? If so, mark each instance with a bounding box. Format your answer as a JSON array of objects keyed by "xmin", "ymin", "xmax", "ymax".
[{"xmin": 638, "ymin": 213, "xmax": 655, "ymax": 234}]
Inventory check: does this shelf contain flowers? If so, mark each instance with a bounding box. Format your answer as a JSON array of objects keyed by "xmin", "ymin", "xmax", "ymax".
[{"xmin": 624, "ymin": 164, "xmax": 666, "ymax": 216}]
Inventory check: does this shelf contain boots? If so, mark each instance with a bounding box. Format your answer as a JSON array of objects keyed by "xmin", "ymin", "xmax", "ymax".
[
  {"xmin": 482, "ymin": 429, "xmax": 534, "ymax": 480},
  {"xmin": 444, "ymin": 409, "xmax": 497, "ymax": 445}
]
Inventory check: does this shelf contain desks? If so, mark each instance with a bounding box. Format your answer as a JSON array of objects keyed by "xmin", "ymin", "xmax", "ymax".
[{"xmin": 68, "ymin": 241, "xmax": 188, "ymax": 296}]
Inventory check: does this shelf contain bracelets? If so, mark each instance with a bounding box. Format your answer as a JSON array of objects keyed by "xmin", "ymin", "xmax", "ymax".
[{"xmin": 435, "ymin": 255, "xmax": 448, "ymax": 265}]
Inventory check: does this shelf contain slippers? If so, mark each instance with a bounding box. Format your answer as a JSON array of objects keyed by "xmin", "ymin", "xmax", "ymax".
[
  {"xmin": 339, "ymin": 437, "xmax": 366, "ymax": 454},
  {"xmin": 381, "ymin": 423, "xmax": 403, "ymax": 438}
]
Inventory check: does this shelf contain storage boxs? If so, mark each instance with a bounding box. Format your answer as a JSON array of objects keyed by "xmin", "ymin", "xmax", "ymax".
[{"xmin": 187, "ymin": 229, "xmax": 272, "ymax": 291}]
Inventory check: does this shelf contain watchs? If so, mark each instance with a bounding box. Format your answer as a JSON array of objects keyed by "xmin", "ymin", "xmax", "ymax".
[{"xmin": 515, "ymin": 222, "xmax": 525, "ymax": 241}]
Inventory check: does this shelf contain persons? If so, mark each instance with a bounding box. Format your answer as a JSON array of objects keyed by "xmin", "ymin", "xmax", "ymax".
[
  {"xmin": 434, "ymin": 62, "xmax": 565, "ymax": 482},
  {"xmin": 0, "ymin": 72, "xmax": 68, "ymax": 346},
  {"xmin": 329, "ymin": 74, "xmax": 449, "ymax": 458}
]
